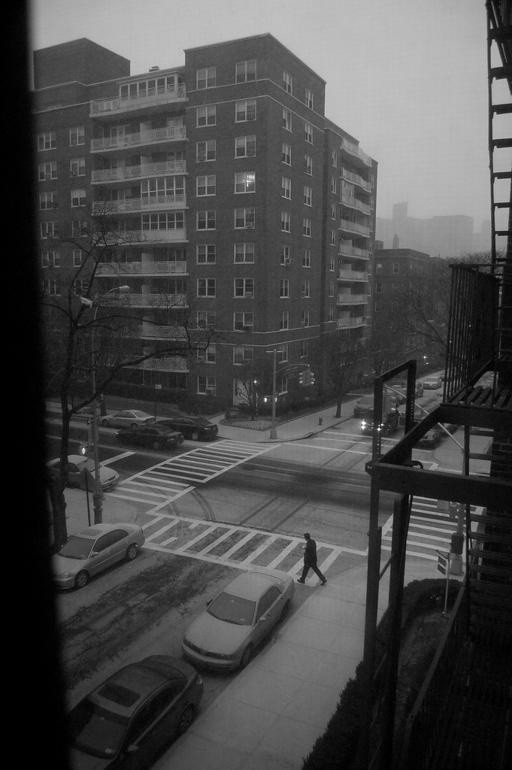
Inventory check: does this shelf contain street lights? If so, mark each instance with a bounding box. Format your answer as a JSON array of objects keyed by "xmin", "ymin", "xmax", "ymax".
[{"xmin": 91, "ymin": 284, "xmax": 129, "ymax": 523}]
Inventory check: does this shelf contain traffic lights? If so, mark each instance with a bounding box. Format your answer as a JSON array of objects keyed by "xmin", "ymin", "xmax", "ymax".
[
  {"xmin": 80, "ymin": 446, "xmax": 88, "ymax": 457},
  {"xmin": 299, "ymin": 371, "xmax": 305, "ymax": 386},
  {"xmin": 263, "ymin": 396, "xmax": 268, "ymax": 405},
  {"xmin": 308, "ymin": 371, "xmax": 315, "ymax": 386}
]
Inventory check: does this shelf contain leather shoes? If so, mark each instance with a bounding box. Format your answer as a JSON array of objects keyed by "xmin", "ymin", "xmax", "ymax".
[
  {"xmin": 320, "ymin": 579, "xmax": 327, "ymax": 585},
  {"xmin": 297, "ymin": 579, "xmax": 305, "ymax": 584}
]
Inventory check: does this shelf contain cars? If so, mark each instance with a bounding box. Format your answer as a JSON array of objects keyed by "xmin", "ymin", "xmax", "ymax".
[
  {"xmin": 98, "ymin": 409, "xmax": 156, "ymax": 430},
  {"xmin": 112, "ymin": 423, "xmax": 185, "ymax": 451},
  {"xmin": 65, "ymin": 655, "xmax": 204, "ymax": 769},
  {"xmin": 48, "ymin": 523, "xmax": 145, "ymax": 590},
  {"xmin": 156, "ymin": 415, "xmax": 218, "ymax": 442},
  {"xmin": 181, "ymin": 567, "xmax": 295, "ymax": 672},
  {"xmin": 46, "ymin": 454, "xmax": 120, "ymax": 493},
  {"xmin": 353, "ymin": 363, "xmax": 498, "ymax": 449}
]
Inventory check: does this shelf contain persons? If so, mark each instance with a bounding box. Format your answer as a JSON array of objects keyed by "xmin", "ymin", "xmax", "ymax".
[{"xmin": 297, "ymin": 533, "xmax": 327, "ymax": 586}]
